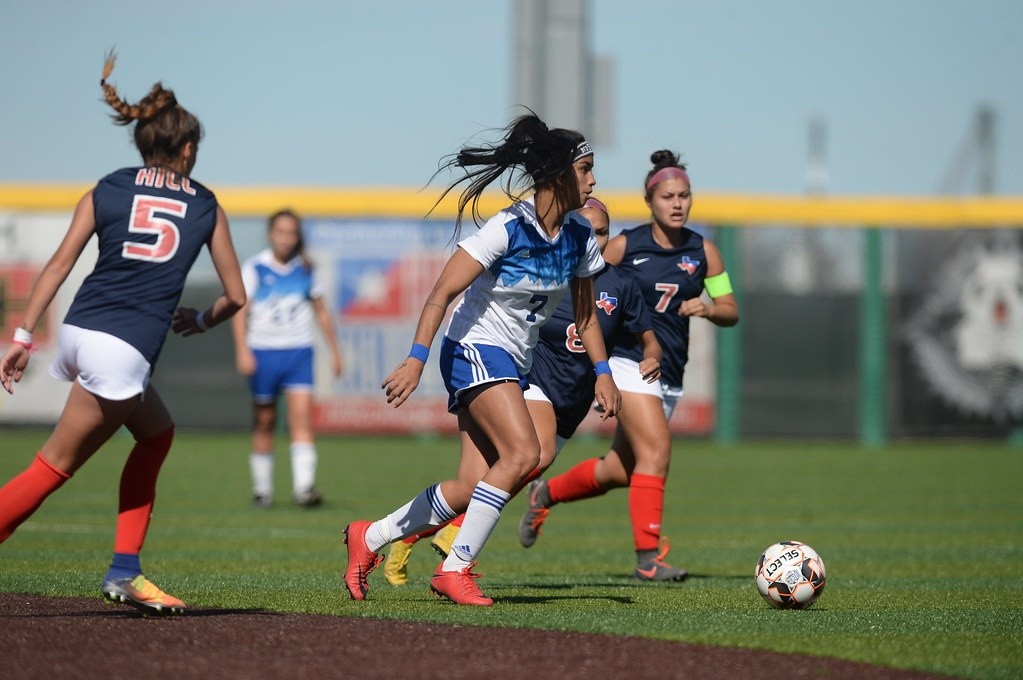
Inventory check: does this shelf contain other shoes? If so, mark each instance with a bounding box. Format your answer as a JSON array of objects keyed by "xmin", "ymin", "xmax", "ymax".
[
  {"xmin": 299, "ymin": 490, "xmax": 324, "ymax": 507},
  {"xmin": 255, "ymin": 495, "xmax": 273, "ymax": 508}
]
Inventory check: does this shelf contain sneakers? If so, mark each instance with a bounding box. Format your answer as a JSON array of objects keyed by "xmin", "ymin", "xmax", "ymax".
[
  {"xmin": 101, "ymin": 573, "xmax": 186, "ymax": 616},
  {"xmin": 518, "ymin": 478, "xmax": 551, "ymax": 547},
  {"xmin": 342, "ymin": 521, "xmax": 385, "ymax": 601},
  {"xmin": 384, "ymin": 540, "xmax": 414, "ymax": 586},
  {"xmin": 633, "ymin": 535, "xmax": 689, "ymax": 582},
  {"xmin": 430, "ymin": 560, "xmax": 493, "ymax": 606},
  {"xmin": 430, "ymin": 522, "xmax": 461, "ymax": 560}
]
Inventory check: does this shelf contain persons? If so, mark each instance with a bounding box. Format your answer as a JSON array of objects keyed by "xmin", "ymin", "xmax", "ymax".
[
  {"xmin": 339, "ymin": 103, "xmax": 738, "ymax": 607},
  {"xmin": 0, "ymin": 46, "xmax": 247, "ymax": 613},
  {"xmin": 232, "ymin": 211, "xmax": 344, "ymax": 505}
]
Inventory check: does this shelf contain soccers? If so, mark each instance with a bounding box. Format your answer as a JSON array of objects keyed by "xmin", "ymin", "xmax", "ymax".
[{"xmin": 754, "ymin": 540, "xmax": 826, "ymax": 610}]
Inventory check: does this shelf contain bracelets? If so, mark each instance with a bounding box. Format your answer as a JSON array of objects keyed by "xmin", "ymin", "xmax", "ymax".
[
  {"xmin": 408, "ymin": 343, "xmax": 430, "ymax": 364},
  {"xmin": 593, "ymin": 361, "xmax": 612, "ymax": 377},
  {"xmin": 12, "ymin": 327, "xmax": 33, "ymax": 349},
  {"xmin": 195, "ymin": 312, "xmax": 210, "ymax": 331}
]
